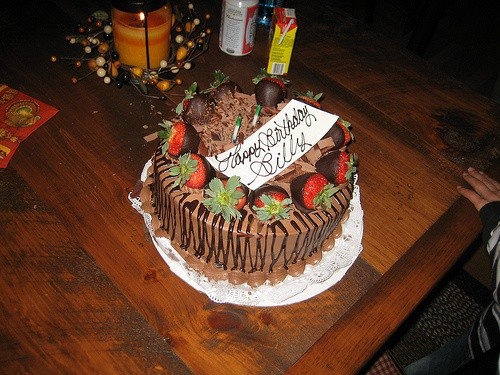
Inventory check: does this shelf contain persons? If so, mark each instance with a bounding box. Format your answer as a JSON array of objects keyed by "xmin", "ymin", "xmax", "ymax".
[{"xmin": 359, "ymin": 166, "xmax": 499, "ymax": 375}]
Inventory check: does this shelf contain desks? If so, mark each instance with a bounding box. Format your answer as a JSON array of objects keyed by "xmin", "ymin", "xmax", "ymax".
[{"xmin": 0, "ymin": 0, "xmax": 500, "ymax": 375}]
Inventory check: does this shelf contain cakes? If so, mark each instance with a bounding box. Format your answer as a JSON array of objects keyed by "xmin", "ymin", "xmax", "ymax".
[{"xmin": 130, "ymin": 69, "xmax": 358, "ymax": 288}]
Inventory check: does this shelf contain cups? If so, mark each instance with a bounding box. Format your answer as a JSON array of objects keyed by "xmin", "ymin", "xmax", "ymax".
[{"xmin": 255, "ymin": 0, "xmax": 283, "ymax": 27}]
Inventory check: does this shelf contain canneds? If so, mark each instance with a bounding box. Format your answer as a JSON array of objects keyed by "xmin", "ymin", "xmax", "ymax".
[{"xmin": 218, "ymin": 0, "xmax": 259, "ymax": 56}]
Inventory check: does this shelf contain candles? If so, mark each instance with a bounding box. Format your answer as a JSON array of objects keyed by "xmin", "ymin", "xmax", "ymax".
[
  {"xmin": 252, "ymin": 104, "xmax": 261, "ymax": 126},
  {"xmin": 233, "ymin": 113, "xmax": 242, "ymax": 142}
]
